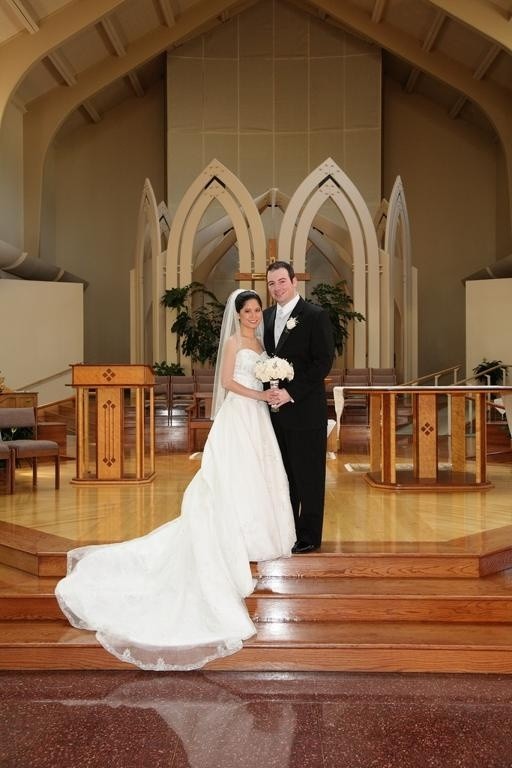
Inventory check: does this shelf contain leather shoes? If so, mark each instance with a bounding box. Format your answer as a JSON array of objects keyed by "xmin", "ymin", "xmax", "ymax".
[{"xmin": 291, "ymin": 541, "xmax": 321, "ymax": 553}]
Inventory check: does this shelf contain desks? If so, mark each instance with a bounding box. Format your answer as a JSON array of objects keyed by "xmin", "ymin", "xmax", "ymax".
[{"xmin": 330, "ymin": 384, "xmax": 511, "ymax": 492}]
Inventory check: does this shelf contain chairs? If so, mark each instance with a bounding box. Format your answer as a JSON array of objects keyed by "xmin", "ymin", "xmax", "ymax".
[
  {"xmin": 0, "ymin": 405, "xmax": 64, "ymax": 495},
  {"xmin": 183, "ymin": 392, "xmax": 215, "ymax": 452},
  {"xmin": 143, "ymin": 367, "xmax": 215, "ymax": 427},
  {"xmin": 323, "ymin": 367, "xmax": 413, "ymax": 428}
]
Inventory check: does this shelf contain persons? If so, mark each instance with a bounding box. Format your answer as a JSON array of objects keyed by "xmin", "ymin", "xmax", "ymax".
[
  {"xmin": 221, "ymin": 288, "xmax": 279, "ymax": 554},
  {"xmin": 260, "ymin": 260, "xmax": 336, "ymax": 555}
]
